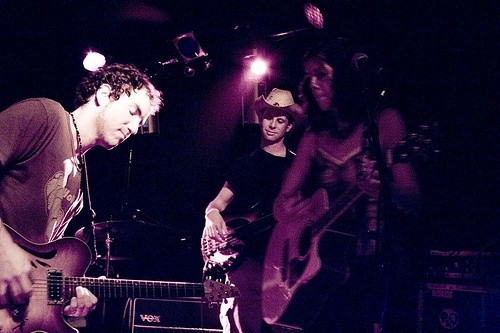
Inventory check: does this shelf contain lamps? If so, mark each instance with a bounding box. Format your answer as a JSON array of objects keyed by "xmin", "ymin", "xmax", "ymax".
[{"xmin": 174, "ymin": 32, "xmax": 209, "ymax": 63}]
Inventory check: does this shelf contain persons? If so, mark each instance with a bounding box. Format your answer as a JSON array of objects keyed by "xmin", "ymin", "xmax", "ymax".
[
  {"xmin": 270, "ymin": 39, "xmax": 423, "ymax": 333},
  {"xmin": 205, "ymin": 88, "xmax": 303, "ymax": 333},
  {"xmin": 0, "ymin": 64, "xmax": 164, "ymax": 333}
]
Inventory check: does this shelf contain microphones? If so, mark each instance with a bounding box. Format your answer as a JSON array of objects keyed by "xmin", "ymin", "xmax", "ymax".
[{"xmin": 352, "ymin": 52, "xmax": 383, "ymax": 75}]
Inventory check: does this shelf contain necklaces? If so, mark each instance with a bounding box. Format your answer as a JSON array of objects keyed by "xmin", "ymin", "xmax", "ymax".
[{"xmin": 69, "ymin": 112, "xmax": 84, "ymax": 165}]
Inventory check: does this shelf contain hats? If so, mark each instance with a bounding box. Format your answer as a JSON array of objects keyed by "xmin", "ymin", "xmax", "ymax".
[{"xmin": 254, "ymin": 88, "xmax": 307, "ymax": 125}]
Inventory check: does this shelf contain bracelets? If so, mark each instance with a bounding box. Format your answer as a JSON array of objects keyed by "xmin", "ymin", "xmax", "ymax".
[{"xmin": 204, "ymin": 209, "xmax": 219, "ymax": 218}]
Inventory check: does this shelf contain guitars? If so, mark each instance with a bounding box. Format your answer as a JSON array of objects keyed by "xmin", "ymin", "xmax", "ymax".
[
  {"xmin": 0, "ymin": 223, "xmax": 241, "ymax": 333},
  {"xmin": 262, "ymin": 121, "xmax": 443, "ymax": 329},
  {"xmin": 202, "ymin": 211, "xmax": 277, "ymax": 271}
]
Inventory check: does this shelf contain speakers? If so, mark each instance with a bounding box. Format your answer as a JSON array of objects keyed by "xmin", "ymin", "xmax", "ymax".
[{"xmin": 131, "ymin": 298, "xmax": 224, "ymax": 333}]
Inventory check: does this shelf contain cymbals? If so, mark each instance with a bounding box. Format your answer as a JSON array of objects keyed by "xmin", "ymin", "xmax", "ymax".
[{"xmin": 75, "ymin": 220, "xmax": 145, "ymax": 260}]
